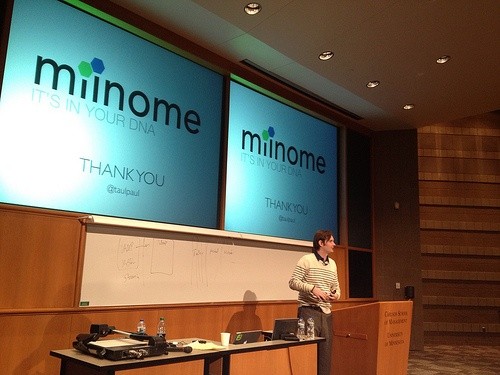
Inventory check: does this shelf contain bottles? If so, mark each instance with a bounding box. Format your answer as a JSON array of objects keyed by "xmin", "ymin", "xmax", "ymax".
[
  {"xmin": 307, "ymin": 316, "xmax": 314, "ymax": 340},
  {"xmin": 157, "ymin": 317, "xmax": 166, "ymax": 339},
  {"xmin": 297, "ymin": 317, "xmax": 305, "ymax": 340},
  {"xmin": 136, "ymin": 320, "xmax": 146, "ymax": 335}
]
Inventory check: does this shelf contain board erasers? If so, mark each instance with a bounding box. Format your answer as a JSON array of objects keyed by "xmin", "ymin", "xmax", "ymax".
[{"xmin": 81, "ymin": 302, "xmax": 89, "ymax": 306}]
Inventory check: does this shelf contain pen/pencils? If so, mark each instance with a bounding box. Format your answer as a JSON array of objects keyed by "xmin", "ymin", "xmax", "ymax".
[{"xmin": 192, "ymin": 341, "xmax": 206, "ymax": 343}]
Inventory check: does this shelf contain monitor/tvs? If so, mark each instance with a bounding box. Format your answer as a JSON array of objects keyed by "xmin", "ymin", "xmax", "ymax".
[{"xmin": 271, "ymin": 318, "xmax": 299, "ymax": 340}]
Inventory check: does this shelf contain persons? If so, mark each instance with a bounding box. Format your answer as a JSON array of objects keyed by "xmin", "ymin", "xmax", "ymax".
[{"xmin": 289, "ymin": 230, "xmax": 341, "ymax": 375}]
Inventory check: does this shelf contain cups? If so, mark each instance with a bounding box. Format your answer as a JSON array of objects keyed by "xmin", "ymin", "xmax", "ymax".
[{"xmin": 220, "ymin": 333, "xmax": 230, "ymax": 348}]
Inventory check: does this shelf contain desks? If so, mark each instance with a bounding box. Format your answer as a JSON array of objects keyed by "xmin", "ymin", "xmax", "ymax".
[{"xmin": 49, "ymin": 330, "xmax": 326, "ymax": 375}]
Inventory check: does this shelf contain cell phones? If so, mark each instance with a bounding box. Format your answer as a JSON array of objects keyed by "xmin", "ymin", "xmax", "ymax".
[{"xmin": 330, "ymin": 290, "xmax": 336, "ymax": 297}]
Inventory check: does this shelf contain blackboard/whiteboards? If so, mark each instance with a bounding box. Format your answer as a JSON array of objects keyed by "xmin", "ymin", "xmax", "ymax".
[{"xmin": 75, "ymin": 225, "xmax": 312, "ymax": 312}]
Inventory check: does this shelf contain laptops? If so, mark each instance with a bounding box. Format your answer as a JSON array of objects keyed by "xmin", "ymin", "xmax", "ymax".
[{"xmin": 233, "ymin": 330, "xmax": 261, "ymax": 344}]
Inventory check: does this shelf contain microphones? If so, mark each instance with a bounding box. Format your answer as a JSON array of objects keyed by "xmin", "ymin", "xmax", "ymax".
[{"xmin": 167, "ymin": 346, "xmax": 192, "ymax": 353}]
